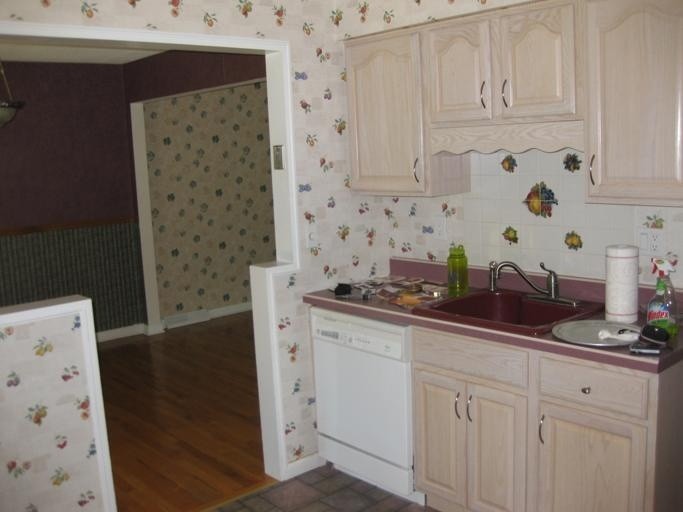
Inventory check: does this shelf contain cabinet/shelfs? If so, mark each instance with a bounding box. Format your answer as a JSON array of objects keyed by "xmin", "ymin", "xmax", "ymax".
[
  {"xmin": 342, "ymin": 28, "xmax": 470, "ymax": 197},
  {"xmin": 577, "ymin": 0, "xmax": 683, "ymax": 207},
  {"xmin": 421, "ymin": 0, "xmax": 583, "ymax": 129},
  {"xmin": 411, "ymin": 322, "xmax": 529, "ymax": 512},
  {"xmin": 529, "ymin": 347, "xmax": 683, "ymax": 512}
]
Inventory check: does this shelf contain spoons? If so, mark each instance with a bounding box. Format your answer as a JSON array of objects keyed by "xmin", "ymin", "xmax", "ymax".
[{"xmin": 597, "ymin": 329, "xmax": 639, "ymax": 342}]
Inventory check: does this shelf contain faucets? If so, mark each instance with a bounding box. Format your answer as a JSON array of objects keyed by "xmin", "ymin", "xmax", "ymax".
[{"xmin": 495, "ymin": 261, "xmax": 560, "ymax": 299}]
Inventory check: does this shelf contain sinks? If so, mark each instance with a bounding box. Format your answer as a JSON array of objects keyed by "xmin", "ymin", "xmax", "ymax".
[{"xmin": 410, "ymin": 286, "xmax": 606, "ymax": 338}]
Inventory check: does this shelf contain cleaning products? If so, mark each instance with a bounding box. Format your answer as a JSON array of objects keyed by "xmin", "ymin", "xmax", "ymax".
[{"xmin": 646, "ymin": 258, "xmax": 678, "ymax": 339}]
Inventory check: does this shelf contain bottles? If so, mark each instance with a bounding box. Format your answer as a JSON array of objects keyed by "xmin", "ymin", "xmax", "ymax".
[{"xmin": 446, "ymin": 245, "xmax": 468, "ymax": 296}]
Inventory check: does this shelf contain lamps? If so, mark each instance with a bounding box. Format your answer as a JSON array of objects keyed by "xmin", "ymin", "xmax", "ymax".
[{"xmin": 0, "ymin": 60, "xmax": 25, "ymax": 127}]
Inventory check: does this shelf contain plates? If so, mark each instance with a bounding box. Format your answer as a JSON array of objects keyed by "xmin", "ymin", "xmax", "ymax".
[{"xmin": 551, "ymin": 321, "xmax": 641, "ymax": 348}]
